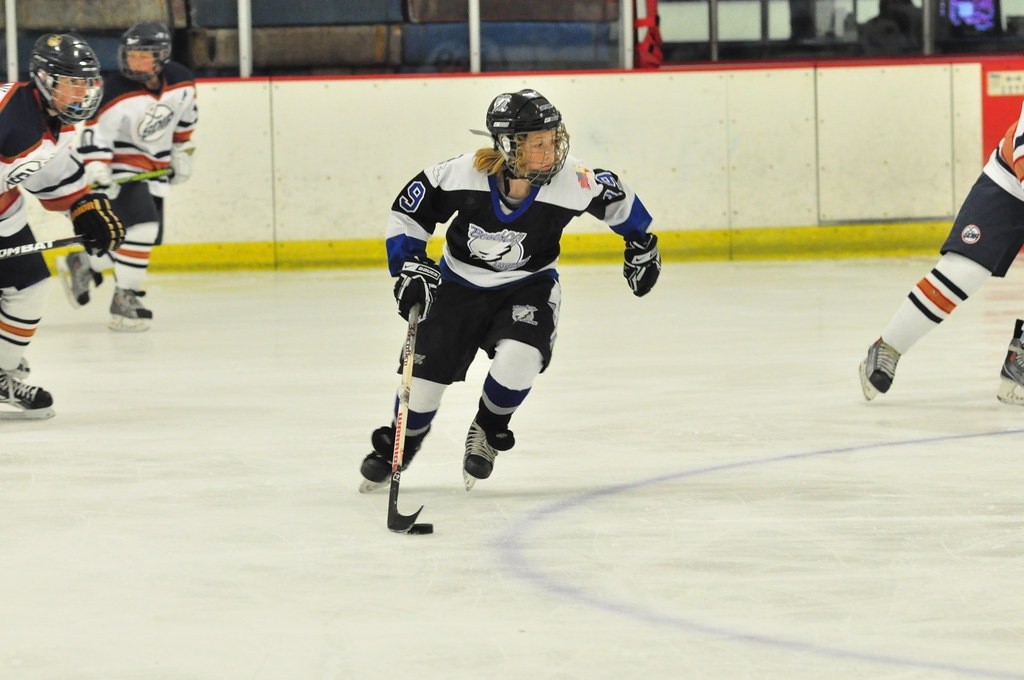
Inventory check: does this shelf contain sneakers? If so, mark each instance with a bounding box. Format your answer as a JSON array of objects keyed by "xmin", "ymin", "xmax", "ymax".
[
  {"xmin": 66, "ymin": 251, "xmax": 104, "ymax": 305},
  {"xmin": 361, "ymin": 423, "xmax": 422, "ymax": 483},
  {"xmin": 109, "ymin": 288, "xmax": 155, "ymax": 319},
  {"xmin": 866, "ymin": 336, "xmax": 902, "ymax": 391},
  {"xmin": 1000, "ymin": 334, "xmax": 1024, "ymax": 385},
  {"xmin": 462, "ymin": 417, "xmax": 516, "ymax": 478},
  {"xmin": 1, "ymin": 368, "xmax": 53, "ymax": 410}
]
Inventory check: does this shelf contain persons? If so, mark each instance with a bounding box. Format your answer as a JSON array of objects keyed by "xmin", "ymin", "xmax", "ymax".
[
  {"xmin": 859, "ymin": 105, "xmax": 1024, "ymax": 407},
  {"xmin": 0, "ymin": 34, "xmax": 127, "ymax": 420},
  {"xmin": 357, "ymin": 90, "xmax": 659, "ymax": 494},
  {"xmin": 56, "ymin": 21, "xmax": 199, "ymax": 332}
]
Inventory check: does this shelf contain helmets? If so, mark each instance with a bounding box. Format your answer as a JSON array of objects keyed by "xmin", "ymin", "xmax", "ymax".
[
  {"xmin": 27, "ymin": 33, "xmax": 105, "ymax": 124},
  {"xmin": 486, "ymin": 90, "xmax": 567, "ymax": 186},
  {"xmin": 116, "ymin": 20, "xmax": 173, "ymax": 77}
]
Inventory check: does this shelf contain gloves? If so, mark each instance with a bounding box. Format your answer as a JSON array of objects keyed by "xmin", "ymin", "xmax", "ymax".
[
  {"xmin": 167, "ymin": 149, "xmax": 194, "ymax": 185},
  {"xmin": 72, "ymin": 191, "xmax": 127, "ymax": 257},
  {"xmin": 623, "ymin": 233, "xmax": 662, "ymax": 299},
  {"xmin": 393, "ymin": 254, "xmax": 445, "ymax": 324}
]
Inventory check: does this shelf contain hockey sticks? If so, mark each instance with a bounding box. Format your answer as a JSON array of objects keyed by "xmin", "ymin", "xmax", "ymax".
[
  {"xmin": 384, "ymin": 303, "xmax": 425, "ymax": 535},
  {"xmin": 0, "ymin": 233, "xmax": 95, "ymax": 261},
  {"xmin": 88, "ymin": 167, "xmax": 173, "ymax": 191}
]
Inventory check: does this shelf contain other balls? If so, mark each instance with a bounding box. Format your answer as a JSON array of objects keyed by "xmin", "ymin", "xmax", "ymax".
[{"xmin": 406, "ymin": 523, "xmax": 434, "ymax": 535}]
